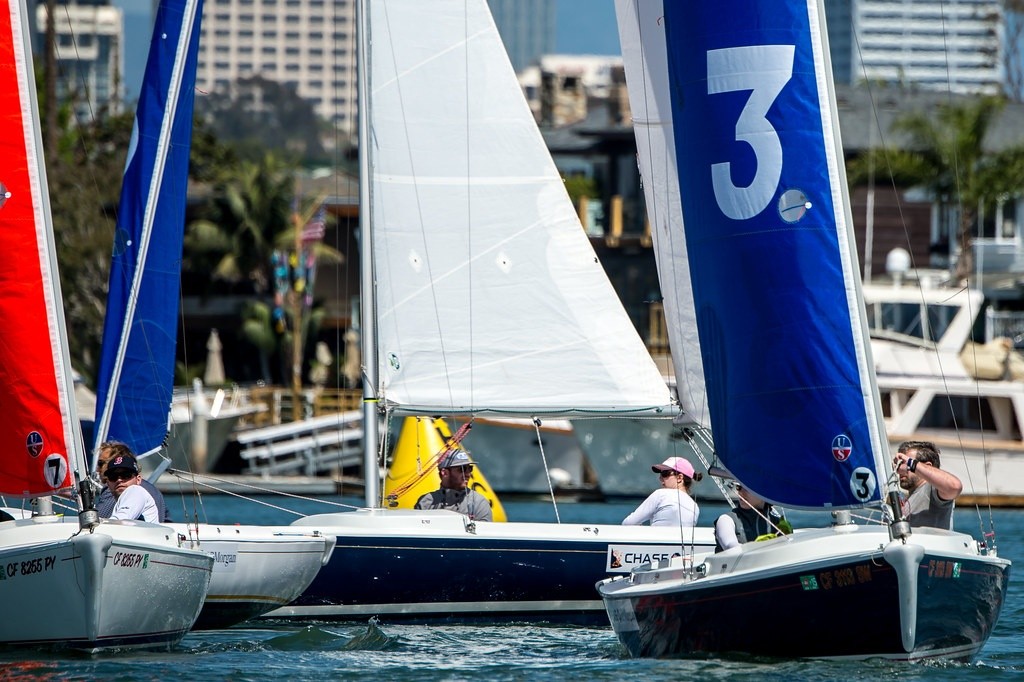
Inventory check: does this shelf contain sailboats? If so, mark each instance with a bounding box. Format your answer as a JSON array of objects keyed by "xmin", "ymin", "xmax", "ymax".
[
  {"xmin": 595, "ymin": 0, "xmax": 1011, "ymax": 663},
  {"xmin": 0, "ymin": 0, "xmax": 339, "ymax": 651},
  {"xmin": 246, "ymin": 0, "xmax": 787, "ymax": 622}
]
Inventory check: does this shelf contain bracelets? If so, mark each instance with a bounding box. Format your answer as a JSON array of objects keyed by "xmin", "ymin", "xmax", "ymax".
[{"xmin": 907, "ymin": 458, "xmax": 920, "ymax": 473}]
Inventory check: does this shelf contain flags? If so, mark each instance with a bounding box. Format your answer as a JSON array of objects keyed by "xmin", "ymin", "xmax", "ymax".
[{"xmin": 302, "ymin": 208, "xmax": 324, "ymax": 248}]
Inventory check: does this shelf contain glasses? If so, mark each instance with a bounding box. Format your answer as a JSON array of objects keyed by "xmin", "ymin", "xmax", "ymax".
[
  {"xmin": 106, "ymin": 470, "xmax": 136, "ymax": 483},
  {"xmin": 449, "ymin": 466, "xmax": 473, "ymax": 473},
  {"xmin": 97, "ymin": 460, "xmax": 108, "ymax": 469},
  {"xmin": 660, "ymin": 471, "xmax": 680, "ymax": 478},
  {"xmin": 736, "ymin": 485, "xmax": 742, "ymax": 490}
]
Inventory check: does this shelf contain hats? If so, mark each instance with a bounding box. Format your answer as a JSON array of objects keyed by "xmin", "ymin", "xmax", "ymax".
[
  {"xmin": 103, "ymin": 457, "xmax": 138, "ymax": 479},
  {"xmin": 438, "ymin": 450, "xmax": 479, "ymax": 470},
  {"xmin": 652, "ymin": 457, "xmax": 695, "ymax": 479}
]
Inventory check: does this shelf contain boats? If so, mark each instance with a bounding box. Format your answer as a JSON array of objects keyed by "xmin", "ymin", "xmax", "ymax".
[{"xmin": 648, "ymin": 189, "xmax": 1024, "ymax": 508}]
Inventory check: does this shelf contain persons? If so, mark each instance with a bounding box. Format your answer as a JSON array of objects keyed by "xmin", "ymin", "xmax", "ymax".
[
  {"xmin": 622, "ymin": 457, "xmax": 702, "ymax": 526},
  {"xmin": 892, "ymin": 441, "xmax": 962, "ymax": 531},
  {"xmin": 104, "ymin": 457, "xmax": 159, "ymax": 524},
  {"xmin": 713, "ymin": 484, "xmax": 794, "ymax": 554},
  {"xmin": 96, "ymin": 442, "xmax": 165, "ymax": 522},
  {"xmin": 414, "ymin": 451, "xmax": 492, "ymax": 522}
]
